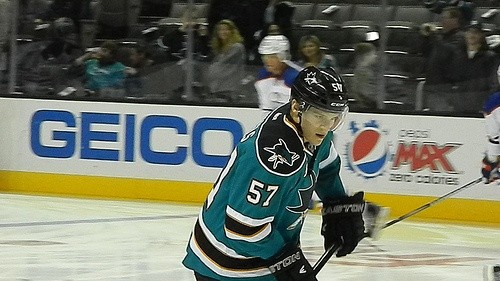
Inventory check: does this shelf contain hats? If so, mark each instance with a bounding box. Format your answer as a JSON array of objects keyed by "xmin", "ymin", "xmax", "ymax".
[{"xmin": 465, "ymin": 20, "xmax": 481, "ymax": 31}]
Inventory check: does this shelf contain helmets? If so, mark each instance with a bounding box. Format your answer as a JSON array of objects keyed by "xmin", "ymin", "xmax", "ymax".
[
  {"xmin": 258, "ymin": 35, "xmax": 291, "ymax": 55},
  {"xmin": 292, "ymin": 66, "xmax": 348, "ymax": 112}
]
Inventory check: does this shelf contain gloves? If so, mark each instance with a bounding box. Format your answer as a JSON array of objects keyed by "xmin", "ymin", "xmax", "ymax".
[
  {"xmin": 267, "ymin": 248, "xmax": 318, "ymax": 281},
  {"xmin": 482, "ymin": 158, "xmax": 499, "ymax": 183},
  {"xmin": 322, "ymin": 192, "xmax": 364, "ymax": 257}
]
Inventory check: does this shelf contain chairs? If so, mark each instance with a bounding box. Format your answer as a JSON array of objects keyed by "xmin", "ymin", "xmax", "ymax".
[{"xmin": 0, "ymin": 0, "xmax": 500, "ymax": 116}]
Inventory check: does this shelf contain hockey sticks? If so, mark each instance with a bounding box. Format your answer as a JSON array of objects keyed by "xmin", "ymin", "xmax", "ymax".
[
  {"xmin": 364, "ymin": 177, "xmax": 484, "ymax": 238},
  {"xmin": 312, "ymin": 236, "xmax": 343, "ymax": 276}
]
